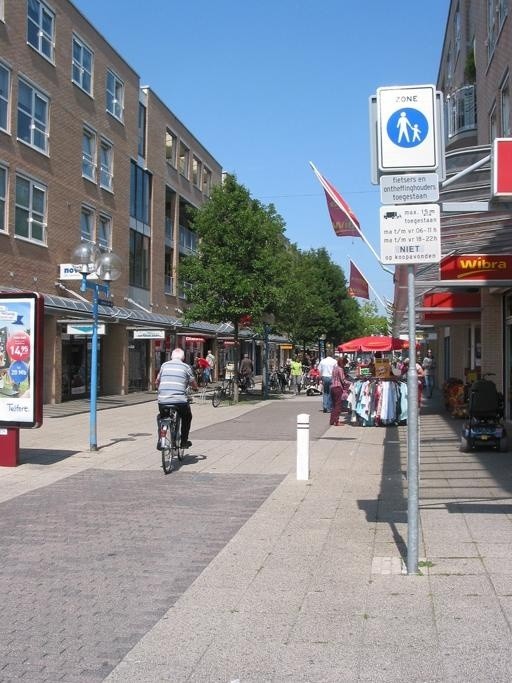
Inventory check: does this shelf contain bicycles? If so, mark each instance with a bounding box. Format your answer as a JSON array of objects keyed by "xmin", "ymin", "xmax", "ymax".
[
  {"xmin": 268, "ymin": 364, "xmax": 316, "ymax": 394},
  {"xmin": 152, "ymin": 388, "xmax": 200, "ymax": 474},
  {"xmin": 212, "ymin": 378, "xmax": 240, "ymax": 409}
]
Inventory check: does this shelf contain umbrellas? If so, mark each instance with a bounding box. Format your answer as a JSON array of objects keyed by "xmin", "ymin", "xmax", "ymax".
[{"xmin": 334, "ymin": 334, "xmax": 409, "ymax": 351}]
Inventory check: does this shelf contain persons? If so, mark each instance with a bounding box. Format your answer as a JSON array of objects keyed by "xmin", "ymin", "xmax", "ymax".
[
  {"xmin": 238, "ymin": 352, "xmax": 254, "ymax": 374},
  {"xmin": 150, "ymin": 346, "xmax": 192, "ymax": 448},
  {"xmin": 318, "ymin": 350, "xmax": 337, "ymax": 412},
  {"xmin": 290, "ymin": 357, "xmax": 302, "ymax": 394},
  {"xmin": 205, "ymin": 349, "xmax": 216, "ymax": 383},
  {"xmin": 422, "ymin": 348, "xmax": 438, "ymax": 399},
  {"xmin": 400, "ymin": 356, "xmax": 424, "ymax": 411},
  {"xmin": 327, "ymin": 357, "xmax": 351, "ymax": 426}
]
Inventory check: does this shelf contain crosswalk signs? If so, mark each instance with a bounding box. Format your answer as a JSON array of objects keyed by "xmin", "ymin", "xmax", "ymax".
[{"xmin": 375, "ymin": 86, "xmax": 440, "ymax": 173}]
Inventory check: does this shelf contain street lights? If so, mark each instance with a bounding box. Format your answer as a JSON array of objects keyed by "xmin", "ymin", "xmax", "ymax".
[{"xmin": 263, "ymin": 311, "xmax": 275, "ymax": 391}]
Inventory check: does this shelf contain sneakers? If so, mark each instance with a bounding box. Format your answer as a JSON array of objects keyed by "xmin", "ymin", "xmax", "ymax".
[
  {"xmin": 180, "ymin": 440, "xmax": 193, "ymax": 449},
  {"xmin": 156, "ymin": 443, "xmax": 169, "ymax": 451}
]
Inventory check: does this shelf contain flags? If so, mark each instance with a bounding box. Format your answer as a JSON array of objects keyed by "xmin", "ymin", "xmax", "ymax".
[
  {"xmin": 348, "ymin": 262, "xmax": 369, "ymax": 299},
  {"xmin": 321, "ymin": 175, "xmax": 361, "ymax": 238}
]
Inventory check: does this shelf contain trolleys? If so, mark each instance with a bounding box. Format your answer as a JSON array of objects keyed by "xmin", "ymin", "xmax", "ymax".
[{"xmin": 458, "ymin": 379, "xmax": 509, "ymax": 453}]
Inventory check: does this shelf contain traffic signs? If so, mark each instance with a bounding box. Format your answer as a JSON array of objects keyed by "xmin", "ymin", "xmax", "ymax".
[{"xmin": 72, "ymin": 243, "xmax": 123, "ymax": 450}]
